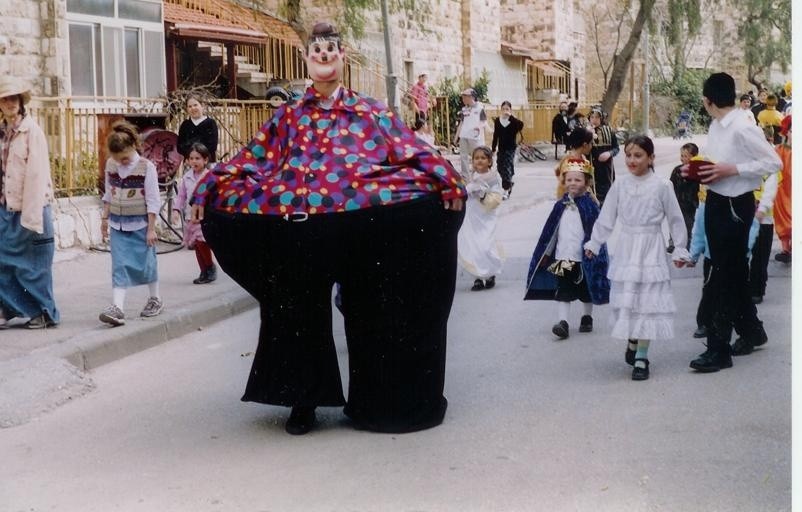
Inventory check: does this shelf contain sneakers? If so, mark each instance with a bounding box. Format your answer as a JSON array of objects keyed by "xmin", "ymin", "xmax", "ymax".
[
  {"xmin": 503, "ymin": 189, "xmax": 511, "ymax": 200},
  {"xmin": 626, "ymin": 339, "xmax": 639, "ymax": 367},
  {"xmin": 207, "ymin": 265, "xmax": 218, "ymax": 282},
  {"xmin": 776, "ymin": 253, "xmax": 791, "ymax": 263},
  {"xmin": 193, "ymin": 272, "xmax": 208, "ymax": 284},
  {"xmin": 485, "ymin": 276, "xmax": 496, "ymax": 289},
  {"xmin": 471, "ymin": 279, "xmax": 485, "ymax": 291},
  {"xmin": 632, "ymin": 358, "xmax": 650, "ymax": 381},
  {"xmin": 581, "ymin": 315, "xmax": 594, "ymax": 332},
  {"xmin": 141, "ymin": 297, "xmax": 164, "ymax": 318},
  {"xmin": 0, "ymin": 304, "xmax": 126, "ymax": 330},
  {"xmin": 553, "ymin": 320, "xmax": 570, "ymax": 338},
  {"xmin": 690, "ymin": 294, "xmax": 768, "ymax": 373}
]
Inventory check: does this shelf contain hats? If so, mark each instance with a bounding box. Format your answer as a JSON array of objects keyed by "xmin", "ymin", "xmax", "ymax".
[
  {"xmin": 461, "ymin": 89, "xmax": 479, "ymax": 97},
  {"xmin": 780, "ymin": 114, "xmax": 792, "ymax": 136},
  {"xmin": 0, "ymin": 76, "xmax": 32, "ymax": 106}
]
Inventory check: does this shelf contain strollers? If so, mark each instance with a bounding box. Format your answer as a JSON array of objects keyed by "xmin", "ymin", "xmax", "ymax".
[{"xmin": 673, "ymin": 110, "xmax": 693, "ymax": 139}]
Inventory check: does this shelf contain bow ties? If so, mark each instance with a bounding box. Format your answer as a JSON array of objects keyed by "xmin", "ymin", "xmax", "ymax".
[{"xmin": 562, "ymin": 113, "xmax": 567, "ymax": 116}]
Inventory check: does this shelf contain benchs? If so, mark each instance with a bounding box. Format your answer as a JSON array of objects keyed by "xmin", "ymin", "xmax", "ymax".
[{"xmin": 551, "ymin": 121, "xmax": 566, "ymax": 160}]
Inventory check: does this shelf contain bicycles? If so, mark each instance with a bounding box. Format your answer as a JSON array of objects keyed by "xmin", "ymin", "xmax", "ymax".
[{"xmin": 516, "ymin": 132, "xmax": 547, "ymax": 162}]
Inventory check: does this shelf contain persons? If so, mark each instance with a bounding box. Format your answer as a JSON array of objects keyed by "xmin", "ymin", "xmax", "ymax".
[
  {"xmin": 747, "ymin": 169, "xmax": 783, "ymax": 304},
  {"xmin": 740, "ymin": 81, "xmax": 792, "ymax": 147},
  {"xmin": 523, "ymin": 157, "xmax": 611, "ymax": 337},
  {"xmin": 176, "ymin": 95, "xmax": 218, "ymax": 170},
  {"xmin": 680, "ymin": 72, "xmax": 784, "ymax": 373},
  {"xmin": 552, "ymin": 101, "xmax": 619, "ymax": 206},
  {"xmin": 188, "ymin": 22, "xmax": 468, "ymax": 433},
  {"xmin": 773, "ymin": 115, "xmax": 792, "ymax": 264},
  {"xmin": 406, "ymin": 74, "xmax": 524, "ymax": 292},
  {"xmin": 686, "ymin": 201, "xmax": 760, "ymax": 338},
  {"xmin": 667, "ymin": 143, "xmax": 700, "ymax": 252},
  {"xmin": 0, "ymin": 73, "xmax": 60, "ymax": 330},
  {"xmin": 583, "ymin": 135, "xmax": 691, "ymax": 379},
  {"xmin": 171, "ymin": 143, "xmax": 217, "ymax": 284},
  {"xmin": 99, "ymin": 120, "xmax": 163, "ymax": 325}
]
